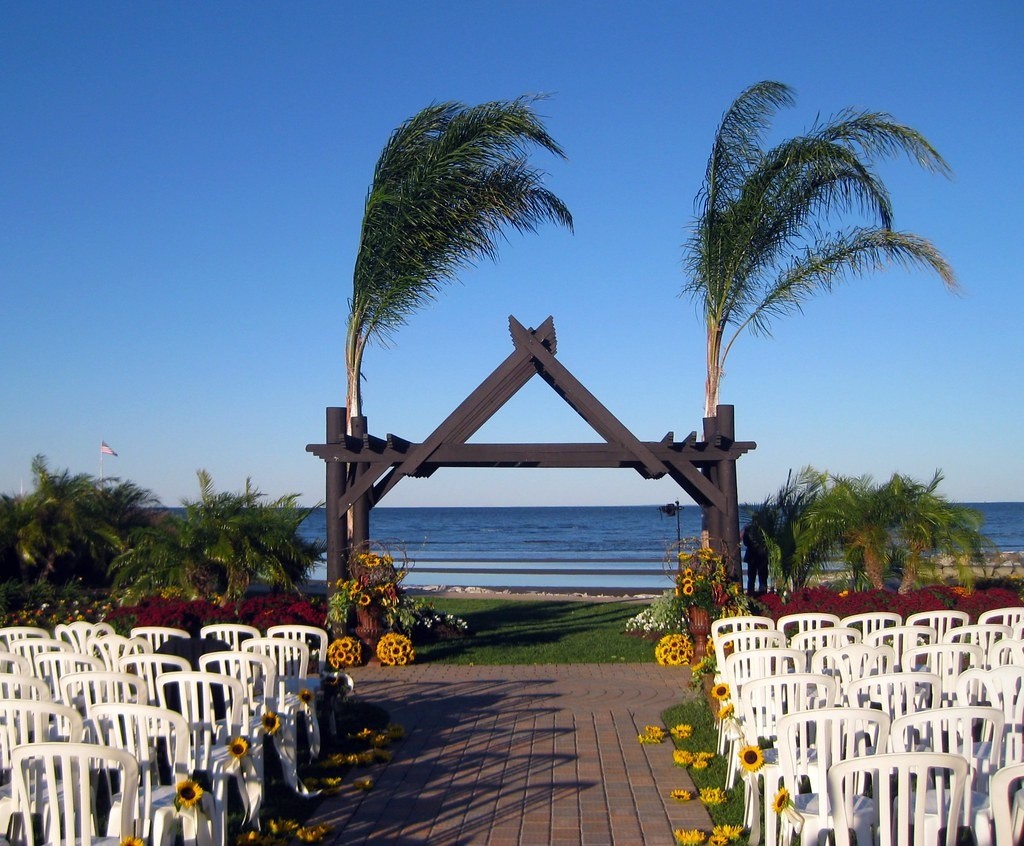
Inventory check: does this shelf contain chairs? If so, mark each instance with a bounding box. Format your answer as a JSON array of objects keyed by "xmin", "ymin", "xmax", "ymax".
[
  {"xmin": 0, "ymin": 621, "xmax": 351, "ymax": 845},
  {"xmin": 710, "ymin": 601, "xmax": 1024, "ymax": 846}
]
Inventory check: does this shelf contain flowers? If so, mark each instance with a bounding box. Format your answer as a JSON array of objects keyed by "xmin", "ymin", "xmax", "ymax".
[
  {"xmin": 624, "ymin": 535, "xmax": 804, "ymax": 846},
  {"xmin": 116, "ymin": 537, "xmax": 469, "ymax": 846}
]
